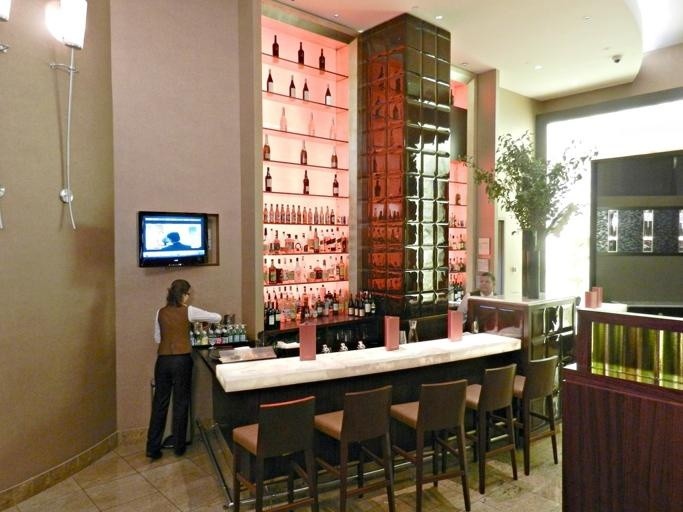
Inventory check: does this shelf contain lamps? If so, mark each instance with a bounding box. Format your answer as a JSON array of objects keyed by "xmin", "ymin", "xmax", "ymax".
[{"xmin": 1, "ymin": 1, "xmax": 91, "ymax": 233}]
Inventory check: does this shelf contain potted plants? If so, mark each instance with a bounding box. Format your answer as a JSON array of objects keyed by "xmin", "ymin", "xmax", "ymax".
[{"xmin": 455, "ymin": 129, "xmax": 600, "ymax": 301}]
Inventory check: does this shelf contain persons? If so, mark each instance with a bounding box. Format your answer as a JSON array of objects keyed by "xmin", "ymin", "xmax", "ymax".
[
  {"xmin": 456, "ymin": 271, "xmax": 497, "ymax": 332},
  {"xmin": 144, "ymin": 279, "xmax": 222, "ymax": 458},
  {"xmin": 159, "ymin": 232, "xmax": 190, "ymax": 251}
]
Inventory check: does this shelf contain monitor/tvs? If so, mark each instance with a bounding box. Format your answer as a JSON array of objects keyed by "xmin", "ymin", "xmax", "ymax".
[{"xmin": 137, "ymin": 210, "xmax": 209, "ymax": 267}]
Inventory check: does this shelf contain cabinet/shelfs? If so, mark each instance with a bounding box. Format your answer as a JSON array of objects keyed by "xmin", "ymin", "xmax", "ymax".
[
  {"xmin": 448, "ymin": 79, "xmax": 469, "ymax": 306},
  {"xmin": 261, "ymin": 28, "xmax": 358, "ymax": 325}
]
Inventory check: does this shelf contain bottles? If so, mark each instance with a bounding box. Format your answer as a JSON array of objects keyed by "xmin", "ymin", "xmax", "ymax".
[
  {"xmin": 263, "ymin": 34, "xmax": 341, "ymax": 197},
  {"xmin": 408, "ymin": 320, "xmax": 419, "ymax": 344},
  {"xmin": 449, "ymin": 191, "xmax": 466, "ymax": 302},
  {"xmin": 263, "ymin": 203, "xmax": 377, "ymax": 329},
  {"xmin": 400, "ymin": 330, "xmax": 406, "ymax": 344},
  {"xmin": 189, "ymin": 321, "xmax": 248, "ymax": 347}
]
